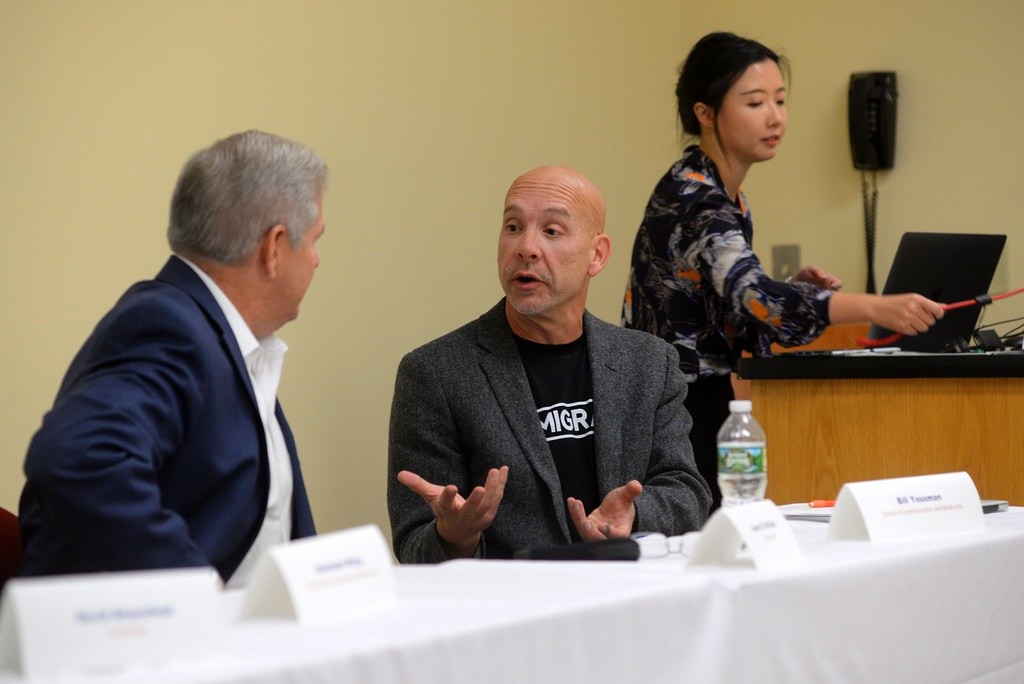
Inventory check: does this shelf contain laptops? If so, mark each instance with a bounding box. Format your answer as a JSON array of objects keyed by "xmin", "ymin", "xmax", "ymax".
[{"xmin": 795, "ymin": 232, "xmax": 1007, "ymax": 356}]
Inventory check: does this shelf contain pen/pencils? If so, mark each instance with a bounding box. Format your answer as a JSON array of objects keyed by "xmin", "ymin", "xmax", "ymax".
[{"xmin": 810, "ymin": 497, "xmax": 837, "ymax": 510}]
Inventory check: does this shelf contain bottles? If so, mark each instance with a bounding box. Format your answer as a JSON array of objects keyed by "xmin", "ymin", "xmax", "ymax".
[{"xmin": 717, "ymin": 398, "xmax": 768, "ymax": 507}]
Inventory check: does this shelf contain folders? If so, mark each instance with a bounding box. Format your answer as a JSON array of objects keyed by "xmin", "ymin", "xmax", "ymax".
[{"xmin": 784, "ymin": 499, "xmax": 1009, "ymax": 528}]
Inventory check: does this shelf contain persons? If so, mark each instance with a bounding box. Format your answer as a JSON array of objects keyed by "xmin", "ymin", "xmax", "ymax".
[
  {"xmin": 19, "ymin": 129, "xmax": 332, "ymax": 595},
  {"xmin": 620, "ymin": 32, "xmax": 950, "ymax": 521},
  {"xmin": 387, "ymin": 162, "xmax": 714, "ymax": 566}
]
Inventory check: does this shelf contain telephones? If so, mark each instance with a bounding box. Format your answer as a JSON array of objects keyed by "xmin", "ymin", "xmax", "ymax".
[{"xmin": 847, "ymin": 69, "xmax": 898, "ymax": 170}]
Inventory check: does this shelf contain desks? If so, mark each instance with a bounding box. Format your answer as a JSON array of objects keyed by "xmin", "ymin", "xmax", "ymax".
[{"xmin": 6, "ymin": 501, "xmax": 1023, "ymax": 682}]
